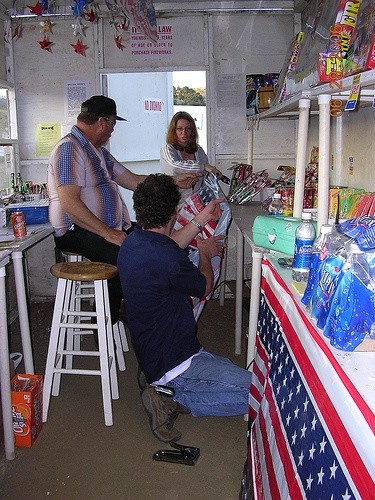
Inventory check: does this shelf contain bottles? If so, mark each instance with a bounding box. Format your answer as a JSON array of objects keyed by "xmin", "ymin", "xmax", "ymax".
[
  {"xmin": 311, "ymin": 219, "xmax": 375, "ymax": 285},
  {"xmin": 293, "ymin": 212, "xmax": 316, "ymax": 282},
  {"xmin": 9, "ymin": 173, "xmax": 24, "ymax": 195},
  {"xmin": 268, "ymin": 194, "xmax": 284, "ymax": 215},
  {"xmin": 0, "ymin": 199, "xmax": 7, "ymax": 229}
]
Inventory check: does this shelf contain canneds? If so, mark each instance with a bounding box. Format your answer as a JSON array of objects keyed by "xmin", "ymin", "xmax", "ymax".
[
  {"xmin": 12, "ymin": 211, "xmax": 27, "ymax": 238},
  {"xmin": 23, "ymin": 181, "xmax": 32, "ymax": 193}
]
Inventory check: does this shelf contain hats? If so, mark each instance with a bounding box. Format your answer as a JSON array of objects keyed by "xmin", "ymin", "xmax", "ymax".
[{"xmin": 81, "ymin": 95, "xmax": 127, "ymax": 121}]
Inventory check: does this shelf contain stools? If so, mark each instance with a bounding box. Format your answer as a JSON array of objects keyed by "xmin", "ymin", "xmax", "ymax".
[{"xmin": 39, "ymin": 251, "xmax": 130, "ymax": 427}]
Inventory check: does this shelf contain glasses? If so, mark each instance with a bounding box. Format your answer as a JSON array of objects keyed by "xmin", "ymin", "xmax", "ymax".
[
  {"xmin": 176, "ymin": 128, "xmax": 192, "ymax": 132},
  {"xmin": 97, "ymin": 115, "xmax": 116, "ymax": 127}
]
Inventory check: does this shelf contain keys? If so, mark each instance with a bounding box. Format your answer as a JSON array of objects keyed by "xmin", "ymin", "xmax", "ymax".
[{"xmin": 150, "ymin": 384, "xmax": 175, "ymax": 398}]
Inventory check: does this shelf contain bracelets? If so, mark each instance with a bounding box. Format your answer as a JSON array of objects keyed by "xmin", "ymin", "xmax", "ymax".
[{"xmin": 190, "ymin": 219, "xmax": 203, "ymax": 231}]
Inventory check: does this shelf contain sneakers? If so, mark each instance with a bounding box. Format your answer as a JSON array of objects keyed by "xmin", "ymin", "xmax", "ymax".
[{"xmin": 137, "ymin": 366, "xmax": 184, "ymax": 444}]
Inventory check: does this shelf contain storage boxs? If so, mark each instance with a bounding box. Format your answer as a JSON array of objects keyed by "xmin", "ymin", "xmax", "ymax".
[
  {"xmin": 3, "ymin": 203, "xmax": 50, "ymax": 224},
  {"xmin": 10, "ymin": 374, "xmax": 43, "ymax": 447},
  {"xmin": 319, "ymin": 0, "xmax": 375, "ymax": 83},
  {"xmin": 251, "ymin": 215, "xmax": 318, "ymax": 254},
  {"xmin": 272, "ymin": 32, "xmax": 305, "ymax": 103}
]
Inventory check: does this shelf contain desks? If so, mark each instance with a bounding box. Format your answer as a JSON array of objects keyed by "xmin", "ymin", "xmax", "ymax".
[
  {"xmin": 0, "ymin": 217, "xmax": 82, "ymax": 461},
  {"xmin": 239, "ymin": 254, "xmax": 375, "ymax": 500},
  {"xmin": 222, "ymin": 198, "xmax": 277, "ymax": 371}
]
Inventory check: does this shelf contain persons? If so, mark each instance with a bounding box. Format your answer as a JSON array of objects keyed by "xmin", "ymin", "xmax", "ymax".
[
  {"xmin": 47, "ymin": 95, "xmax": 203, "ymax": 353},
  {"xmin": 117, "ymin": 174, "xmax": 252, "ymax": 444},
  {"xmin": 160, "ymin": 111, "xmax": 222, "ymax": 205}
]
